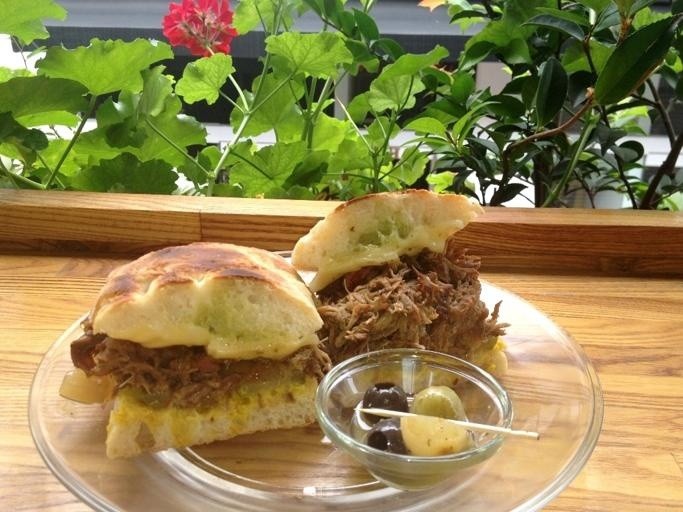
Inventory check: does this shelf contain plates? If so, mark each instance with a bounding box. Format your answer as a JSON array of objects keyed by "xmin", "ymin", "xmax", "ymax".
[{"xmin": 26, "ymin": 250, "xmax": 606, "ymax": 511}]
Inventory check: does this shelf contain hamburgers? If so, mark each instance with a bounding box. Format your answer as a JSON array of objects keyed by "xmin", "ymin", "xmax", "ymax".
[
  {"xmin": 59, "ymin": 239, "xmax": 332, "ymax": 459},
  {"xmin": 290, "ymin": 188, "xmax": 511, "ymax": 408}
]
